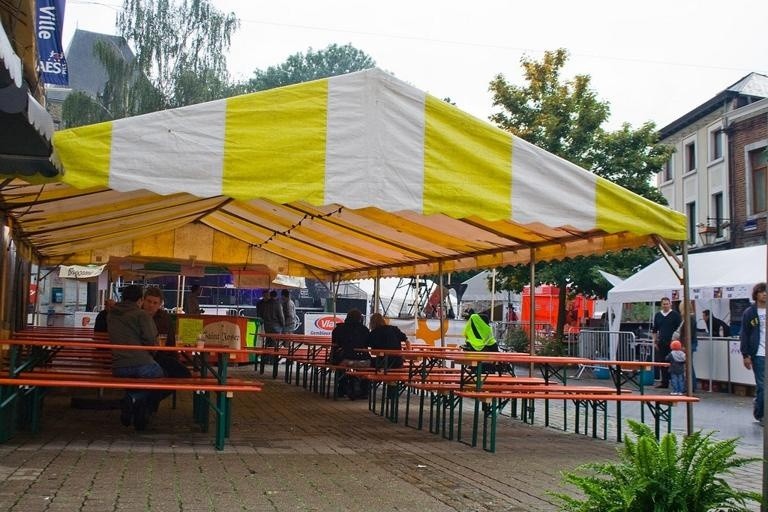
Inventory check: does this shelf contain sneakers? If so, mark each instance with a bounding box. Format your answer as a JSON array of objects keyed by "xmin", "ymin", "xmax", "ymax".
[
  {"xmin": 134, "ymin": 402, "xmax": 146, "ymax": 431},
  {"xmin": 121, "ymin": 396, "xmax": 132, "ymax": 426},
  {"xmin": 670, "ymin": 392, "xmax": 683, "ymax": 396}
]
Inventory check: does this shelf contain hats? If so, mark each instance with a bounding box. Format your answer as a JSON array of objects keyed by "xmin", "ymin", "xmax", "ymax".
[{"xmin": 670, "ymin": 341, "xmax": 681, "ymax": 350}]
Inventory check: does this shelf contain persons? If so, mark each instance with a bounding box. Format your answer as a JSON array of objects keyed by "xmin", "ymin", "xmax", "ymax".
[
  {"xmin": 366, "ymin": 312, "xmax": 414, "ymax": 371},
  {"xmin": 96, "ymin": 297, "xmax": 118, "ymax": 335},
  {"xmin": 255, "ymin": 287, "xmax": 270, "ymax": 318},
  {"xmin": 185, "ymin": 284, "xmax": 206, "ymax": 318},
  {"xmin": 701, "ymin": 310, "xmax": 731, "ymax": 338},
  {"xmin": 107, "ymin": 285, "xmax": 166, "ymax": 429},
  {"xmin": 262, "ymin": 290, "xmax": 287, "ymax": 364},
  {"xmin": 281, "ymin": 289, "xmax": 302, "ymax": 365},
  {"xmin": 462, "ymin": 303, "xmax": 474, "ymax": 319},
  {"xmin": 142, "ymin": 285, "xmax": 187, "ymax": 421},
  {"xmin": 740, "ymin": 282, "xmax": 767, "ymax": 428},
  {"xmin": 650, "ymin": 297, "xmax": 683, "ymax": 390},
  {"xmin": 330, "ymin": 309, "xmax": 371, "ymax": 366},
  {"xmin": 505, "ymin": 302, "xmax": 519, "ymax": 322},
  {"xmin": 664, "ymin": 339, "xmax": 689, "ymax": 396},
  {"xmin": 670, "ymin": 299, "xmax": 701, "ymax": 394}
]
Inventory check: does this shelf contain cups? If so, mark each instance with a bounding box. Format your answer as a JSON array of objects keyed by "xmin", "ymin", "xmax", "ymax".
[
  {"xmin": 194, "ymin": 333, "xmax": 205, "ymax": 349},
  {"xmin": 157, "ymin": 333, "xmax": 168, "ymax": 347},
  {"xmin": 174, "ymin": 334, "xmax": 185, "ymax": 347}
]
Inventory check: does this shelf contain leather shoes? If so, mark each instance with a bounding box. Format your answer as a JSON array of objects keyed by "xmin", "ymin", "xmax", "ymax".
[{"xmin": 655, "ymin": 383, "xmax": 669, "ymax": 388}]
